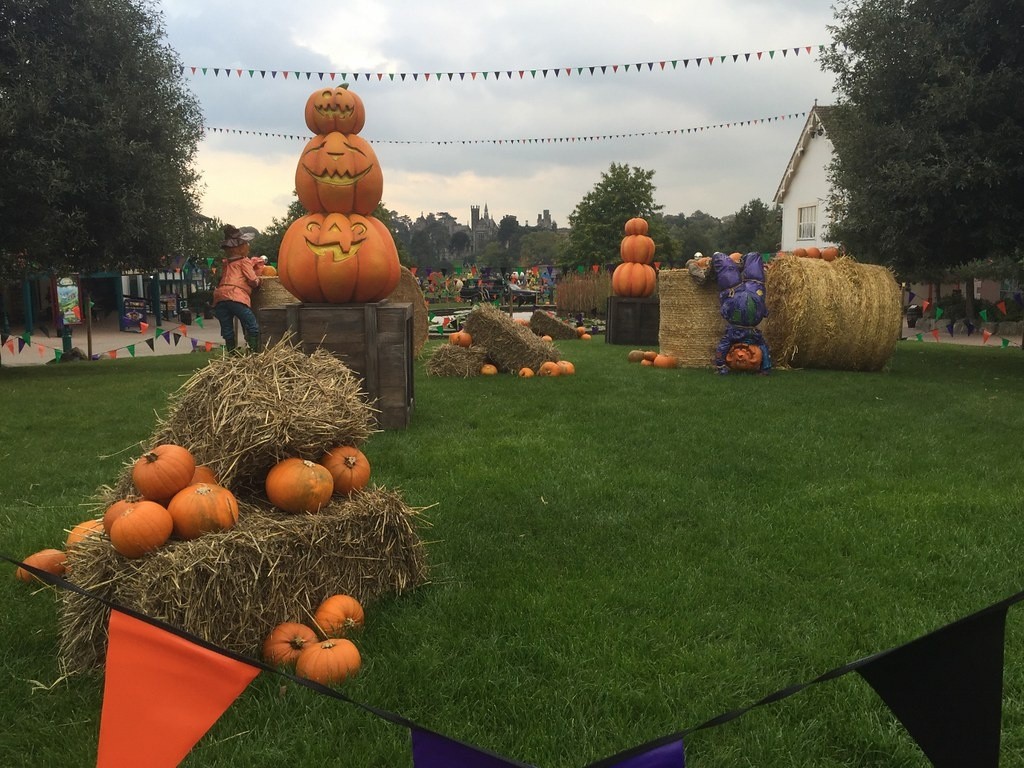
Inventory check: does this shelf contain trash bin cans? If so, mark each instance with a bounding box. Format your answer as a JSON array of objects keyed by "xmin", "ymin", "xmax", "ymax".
[
  {"xmin": 907, "ymin": 305, "xmax": 923, "ymax": 328},
  {"xmin": 180, "ymin": 309, "xmax": 192, "ymax": 325},
  {"xmin": 204, "ymin": 305, "xmax": 213, "ymax": 319}
]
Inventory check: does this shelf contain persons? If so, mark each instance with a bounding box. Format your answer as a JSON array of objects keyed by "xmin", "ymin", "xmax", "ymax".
[
  {"xmin": 709, "ymin": 252, "xmax": 771, "ymax": 378},
  {"xmin": 213, "ymin": 225, "xmax": 265, "ymax": 360}
]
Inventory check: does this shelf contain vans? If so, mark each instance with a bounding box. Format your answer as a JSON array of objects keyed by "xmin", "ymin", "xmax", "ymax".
[{"xmin": 459, "ymin": 279, "xmax": 527, "ymax": 304}]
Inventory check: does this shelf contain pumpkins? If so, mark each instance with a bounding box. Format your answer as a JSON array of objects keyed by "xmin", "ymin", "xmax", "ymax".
[{"xmin": 15, "ymin": 82, "xmax": 838, "ymax": 683}]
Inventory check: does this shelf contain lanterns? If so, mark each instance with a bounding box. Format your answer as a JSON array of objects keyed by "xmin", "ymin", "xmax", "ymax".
[
  {"xmin": 276, "ymin": 210, "xmax": 403, "ymax": 305},
  {"xmin": 305, "ymin": 82, "xmax": 366, "ymax": 136},
  {"xmin": 293, "ymin": 130, "xmax": 384, "ymax": 215}
]
